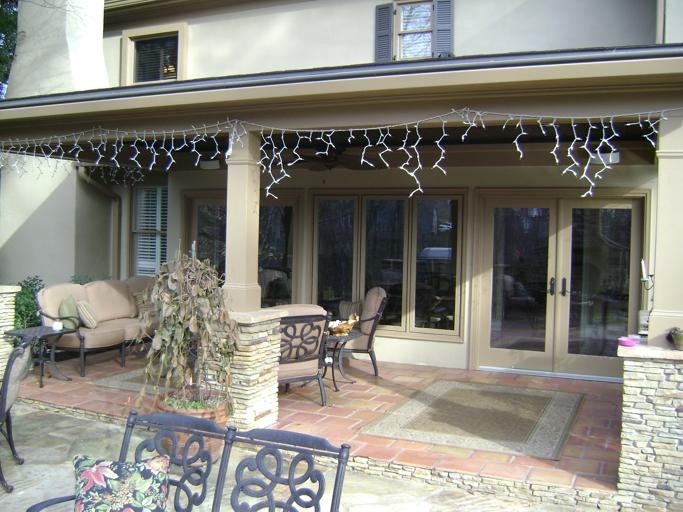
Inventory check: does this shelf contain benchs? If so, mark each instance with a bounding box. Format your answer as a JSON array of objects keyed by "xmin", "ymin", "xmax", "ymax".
[{"xmin": 26, "ymin": 411, "xmax": 351, "ymax": 512}]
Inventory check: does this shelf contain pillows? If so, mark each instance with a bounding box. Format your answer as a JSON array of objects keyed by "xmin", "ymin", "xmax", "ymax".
[
  {"xmin": 58, "ymin": 296, "xmax": 81, "ymax": 328},
  {"xmin": 77, "ymin": 298, "xmax": 98, "ymax": 329},
  {"xmin": 72, "ymin": 454, "xmax": 170, "ymax": 512},
  {"xmin": 339, "ymin": 300, "xmax": 363, "ymax": 329}
]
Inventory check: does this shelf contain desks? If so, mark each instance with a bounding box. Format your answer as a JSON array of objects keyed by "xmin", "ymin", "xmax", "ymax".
[
  {"xmin": 4, "ymin": 326, "xmax": 76, "ymax": 388},
  {"xmin": 302, "ymin": 331, "xmax": 364, "ymax": 391}
]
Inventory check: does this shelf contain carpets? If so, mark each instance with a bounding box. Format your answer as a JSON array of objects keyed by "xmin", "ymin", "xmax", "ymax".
[
  {"xmin": 357, "ymin": 379, "xmax": 587, "ymax": 460},
  {"xmin": 84, "ymin": 367, "xmax": 206, "ymax": 395}
]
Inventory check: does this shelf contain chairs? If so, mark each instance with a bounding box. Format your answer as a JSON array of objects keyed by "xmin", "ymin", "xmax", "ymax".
[
  {"xmin": 0, "ymin": 342, "xmax": 33, "ymax": 493},
  {"xmin": 264, "ymin": 304, "xmax": 329, "ymax": 407},
  {"xmin": 323, "ymin": 286, "xmax": 389, "ymax": 376},
  {"xmin": 499, "ymin": 275, "xmax": 536, "ymax": 327}
]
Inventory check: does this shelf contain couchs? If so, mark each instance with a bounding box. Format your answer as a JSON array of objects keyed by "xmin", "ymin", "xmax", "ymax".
[{"xmin": 36, "ymin": 277, "xmax": 154, "ymax": 377}]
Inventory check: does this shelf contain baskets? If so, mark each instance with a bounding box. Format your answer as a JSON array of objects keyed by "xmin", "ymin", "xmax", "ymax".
[{"xmin": 329, "ymin": 324, "xmax": 353, "ymax": 336}]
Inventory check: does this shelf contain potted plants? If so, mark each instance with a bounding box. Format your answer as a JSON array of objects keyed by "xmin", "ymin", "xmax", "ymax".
[{"xmin": 134, "ymin": 239, "xmax": 242, "ymax": 466}]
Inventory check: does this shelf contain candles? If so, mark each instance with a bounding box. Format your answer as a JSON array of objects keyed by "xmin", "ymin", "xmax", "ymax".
[{"xmin": 641, "ymin": 257, "xmax": 647, "ymax": 279}]
